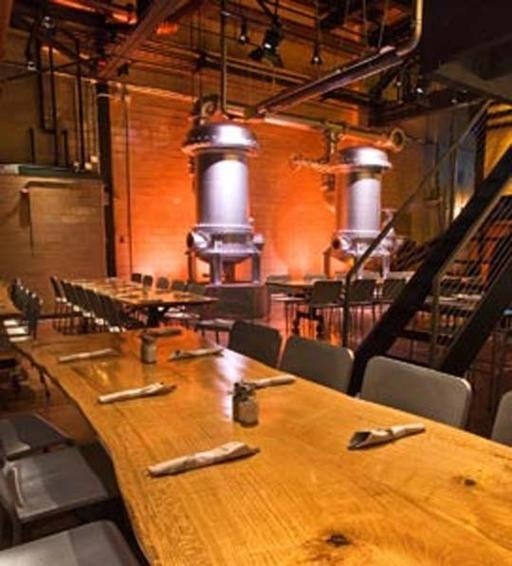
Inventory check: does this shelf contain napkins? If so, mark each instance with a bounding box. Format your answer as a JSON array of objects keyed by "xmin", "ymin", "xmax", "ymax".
[
  {"xmin": 56, "ymin": 347, "xmax": 116, "ymax": 364},
  {"xmin": 97, "ymin": 381, "xmax": 177, "ymax": 403},
  {"xmin": 147, "ymin": 441, "xmax": 261, "ymax": 478},
  {"xmin": 251, "ymin": 376, "xmax": 297, "ymax": 388},
  {"xmin": 169, "ymin": 344, "xmax": 224, "ymax": 362},
  {"xmin": 347, "ymin": 424, "xmax": 425, "ymax": 450}
]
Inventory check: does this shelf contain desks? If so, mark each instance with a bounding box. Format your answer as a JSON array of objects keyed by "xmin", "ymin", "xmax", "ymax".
[
  {"xmin": 17, "ymin": 327, "xmax": 512, "ymax": 566},
  {"xmin": 65, "ymin": 277, "xmax": 217, "ymax": 325}
]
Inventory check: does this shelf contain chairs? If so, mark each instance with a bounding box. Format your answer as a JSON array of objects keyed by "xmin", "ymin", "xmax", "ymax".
[
  {"xmin": 285, "ymin": 274, "xmax": 485, "ymax": 336},
  {"xmin": 0, "ymin": 520, "xmax": 137, "ymax": 566},
  {"xmin": 128, "ymin": 274, "xmax": 267, "ymax": 344},
  {"xmin": 279, "ymin": 335, "xmax": 354, "ymax": 395},
  {"xmin": 0, "ymin": 446, "xmax": 108, "ymax": 546},
  {"xmin": 492, "ymin": 391, "xmax": 510, "ymax": 445},
  {"xmin": 1, "ymin": 410, "xmax": 70, "ymax": 462},
  {"xmin": 52, "ymin": 275, "xmax": 147, "ymax": 334},
  {"xmin": 361, "ymin": 356, "xmax": 472, "ymax": 430},
  {"xmin": 2, "ymin": 279, "xmax": 50, "ymax": 400},
  {"xmin": 226, "ymin": 320, "xmax": 282, "ymax": 368}
]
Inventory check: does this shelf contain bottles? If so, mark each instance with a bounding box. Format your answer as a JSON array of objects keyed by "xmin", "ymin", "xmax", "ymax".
[
  {"xmin": 139, "ymin": 332, "xmax": 159, "ymax": 364},
  {"xmin": 232, "ymin": 382, "xmax": 259, "ymax": 424}
]
